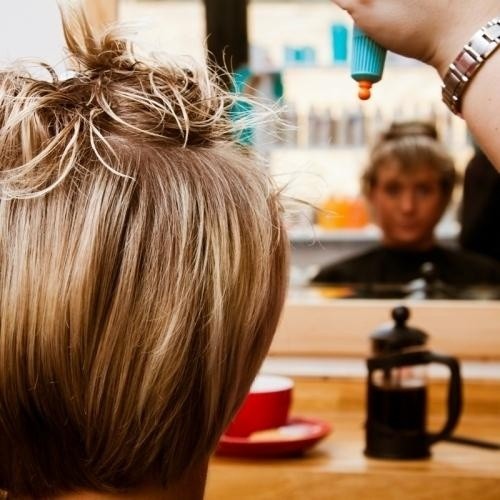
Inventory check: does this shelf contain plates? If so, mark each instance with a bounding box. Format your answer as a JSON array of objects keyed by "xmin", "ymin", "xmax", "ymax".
[{"xmin": 215, "ymin": 416, "xmax": 332, "ymax": 458}]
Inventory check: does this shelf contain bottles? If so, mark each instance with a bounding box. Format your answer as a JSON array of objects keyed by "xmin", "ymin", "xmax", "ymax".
[
  {"xmin": 329, "ymin": 20, "xmax": 349, "ymax": 62},
  {"xmin": 363, "ymin": 305, "xmax": 465, "ymax": 460}
]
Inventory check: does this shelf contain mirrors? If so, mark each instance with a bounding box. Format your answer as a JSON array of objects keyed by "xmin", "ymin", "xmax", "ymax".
[{"xmin": 204, "ymin": 0, "xmax": 500, "ymax": 362}]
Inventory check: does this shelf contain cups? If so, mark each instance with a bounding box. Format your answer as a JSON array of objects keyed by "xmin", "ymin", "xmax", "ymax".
[{"xmin": 225, "ymin": 373, "xmax": 297, "ymax": 437}]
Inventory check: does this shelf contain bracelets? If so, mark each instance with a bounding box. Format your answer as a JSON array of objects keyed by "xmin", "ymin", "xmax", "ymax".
[{"xmin": 438, "ymin": 14, "xmax": 500, "ymax": 116}]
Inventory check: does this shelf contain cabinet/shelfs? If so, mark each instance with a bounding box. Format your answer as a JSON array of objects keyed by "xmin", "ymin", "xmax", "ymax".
[{"xmin": 262, "ymin": 61, "xmax": 463, "ymax": 245}]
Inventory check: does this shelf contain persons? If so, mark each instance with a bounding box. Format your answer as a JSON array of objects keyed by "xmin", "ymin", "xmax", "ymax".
[
  {"xmin": 331, "ymin": 1, "xmax": 500, "ymax": 174},
  {"xmin": 1, "ymin": 0, "xmax": 294, "ymax": 499},
  {"xmin": 310, "ymin": 112, "xmax": 499, "ymax": 300}
]
